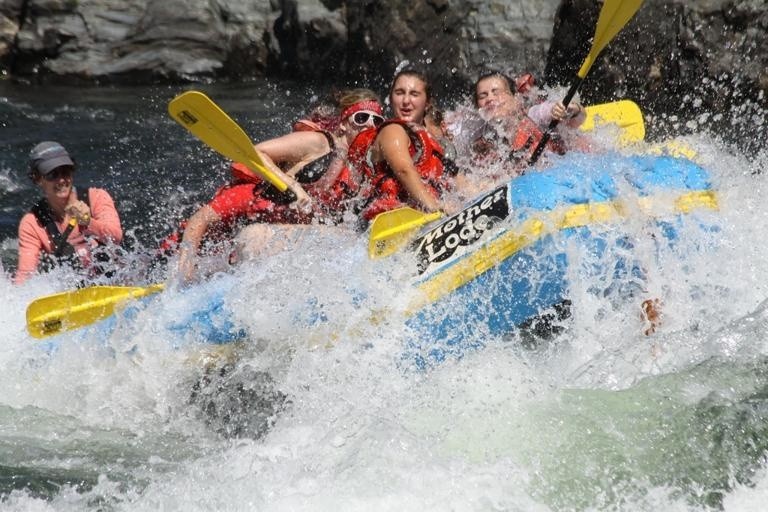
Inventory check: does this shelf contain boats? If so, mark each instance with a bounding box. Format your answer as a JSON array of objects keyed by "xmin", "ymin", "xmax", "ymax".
[{"xmin": 39, "ymin": 149, "xmax": 718, "ymax": 448}]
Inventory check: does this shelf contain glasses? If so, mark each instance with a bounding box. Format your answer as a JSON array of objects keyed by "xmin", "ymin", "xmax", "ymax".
[
  {"xmin": 349, "ymin": 111, "xmax": 386, "ymax": 129},
  {"xmin": 42, "ymin": 170, "xmax": 71, "ymax": 180}
]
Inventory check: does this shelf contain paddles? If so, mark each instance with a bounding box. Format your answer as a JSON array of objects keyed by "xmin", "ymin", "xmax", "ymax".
[
  {"xmin": 369, "ymin": 205, "xmax": 448, "ymax": 257},
  {"xmin": 523, "ymin": 0, "xmax": 644, "ymax": 175},
  {"xmin": 169, "ymin": 92, "xmax": 326, "ymax": 226},
  {"xmin": 577, "ymin": 99, "xmax": 645, "ymax": 145},
  {"xmin": 26, "ymin": 266, "xmax": 231, "ymax": 339},
  {"xmin": 647, "ymin": 139, "xmax": 697, "ymax": 160}
]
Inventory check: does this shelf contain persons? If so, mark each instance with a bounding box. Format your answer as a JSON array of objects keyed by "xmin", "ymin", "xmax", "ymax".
[
  {"xmin": 149, "ymin": 88, "xmax": 385, "ymax": 285},
  {"xmin": 12, "ymin": 141, "xmax": 124, "ymax": 286},
  {"xmin": 357, "ymin": 70, "xmax": 464, "ymax": 224},
  {"xmin": 463, "ymin": 73, "xmax": 597, "ymax": 181}
]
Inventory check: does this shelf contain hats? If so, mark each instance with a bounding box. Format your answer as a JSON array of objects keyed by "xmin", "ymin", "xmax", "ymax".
[{"xmin": 27, "ymin": 142, "xmax": 75, "ymax": 176}]
